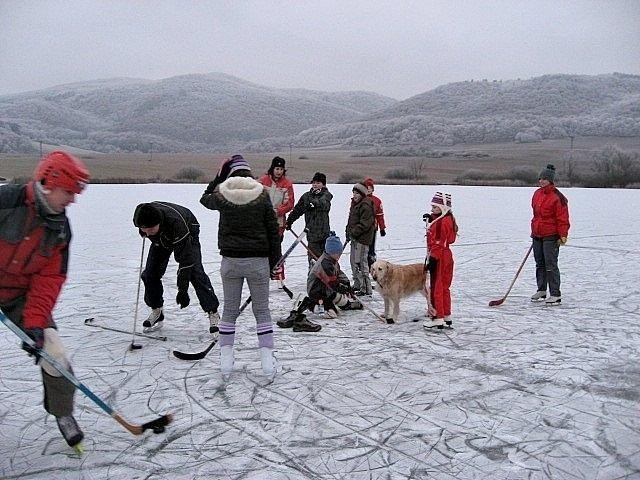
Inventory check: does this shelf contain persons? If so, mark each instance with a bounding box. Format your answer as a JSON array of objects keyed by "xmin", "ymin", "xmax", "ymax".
[
  {"xmin": 285, "ymin": 172, "xmax": 333, "ymax": 263},
  {"xmin": 255, "ymin": 156, "xmax": 294, "ymax": 241},
  {"xmin": 307, "ymin": 237, "xmax": 363, "ymax": 313},
  {"xmin": 133, "ymin": 201, "xmax": 220, "ymax": 335},
  {"xmin": 530, "ymin": 164, "xmax": 570, "ymax": 304},
  {"xmin": 200, "ymin": 155, "xmax": 282, "ymax": 382},
  {"xmin": 1, "ymin": 151, "xmax": 83, "ymax": 456},
  {"xmin": 363, "ymin": 177, "xmax": 386, "ymax": 273},
  {"xmin": 424, "ymin": 192, "xmax": 457, "ymax": 329},
  {"xmin": 346, "ymin": 182, "xmax": 375, "ymax": 295}
]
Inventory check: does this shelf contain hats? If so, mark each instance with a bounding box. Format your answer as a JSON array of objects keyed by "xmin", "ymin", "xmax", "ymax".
[
  {"xmin": 431, "ymin": 191, "xmax": 451, "ymax": 216},
  {"xmin": 133, "ymin": 204, "xmax": 163, "ymax": 228},
  {"xmin": 313, "ymin": 172, "xmax": 326, "ymax": 186},
  {"xmin": 539, "ymin": 164, "xmax": 556, "ymax": 184},
  {"xmin": 353, "ymin": 176, "xmax": 374, "ymax": 199},
  {"xmin": 272, "ymin": 156, "xmax": 285, "ymax": 167},
  {"xmin": 325, "ymin": 236, "xmax": 343, "ymax": 254},
  {"xmin": 227, "ymin": 155, "xmax": 253, "ymax": 177}
]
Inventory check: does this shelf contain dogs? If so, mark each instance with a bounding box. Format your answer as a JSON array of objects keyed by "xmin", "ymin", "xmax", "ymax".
[{"xmin": 371, "ymin": 260, "xmax": 436, "ymax": 324}]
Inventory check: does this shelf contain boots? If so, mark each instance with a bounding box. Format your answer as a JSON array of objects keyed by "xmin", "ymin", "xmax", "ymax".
[
  {"xmin": 143, "ymin": 306, "xmax": 165, "ymax": 327},
  {"xmin": 209, "ymin": 310, "xmax": 220, "ymax": 333},
  {"xmin": 261, "ymin": 347, "xmax": 282, "ymax": 374},
  {"xmin": 221, "ymin": 346, "xmax": 234, "ymax": 372}
]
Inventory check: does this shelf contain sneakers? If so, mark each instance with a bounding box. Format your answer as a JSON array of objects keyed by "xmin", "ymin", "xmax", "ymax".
[
  {"xmin": 352, "ymin": 286, "xmax": 372, "ymax": 297},
  {"xmin": 57, "ymin": 415, "xmax": 84, "ymax": 447},
  {"xmin": 423, "ymin": 315, "xmax": 451, "ymax": 329},
  {"xmin": 338, "ymin": 300, "xmax": 363, "ymax": 310},
  {"xmin": 276, "ymin": 296, "xmax": 321, "ymax": 332},
  {"xmin": 531, "ymin": 291, "xmax": 561, "ymax": 303}
]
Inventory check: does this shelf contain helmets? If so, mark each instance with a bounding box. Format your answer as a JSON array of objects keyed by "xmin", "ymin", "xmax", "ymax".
[{"xmin": 33, "ymin": 151, "xmax": 89, "ymax": 194}]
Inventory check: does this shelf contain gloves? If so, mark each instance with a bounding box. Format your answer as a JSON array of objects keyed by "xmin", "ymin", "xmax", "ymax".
[
  {"xmin": 423, "ymin": 256, "xmax": 438, "ymax": 271},
  {"xmin": 214, "ymin": 159, "xmax": 231, "ymax": 184},
  {"xmin": 380, "ymin": 230, "xmax": 385, "ymax": 236},
  {"xmin": 22, "ymin": 328, "xmax": 43, "ymax": 364},
  {"xmin": 557, "ymin": 237, "xmax": 567, "ymax": 245},
  {"xmin": 269, "ymin": 255, "xmax": 284, "ymax": 280},
  {"xmin": 304, "ymin": 201, "xmax": 316, "ymax": 208},
  {"xmin": 285, "ymin": 222, "xmax": 291, "ymax": 229},
  {"xmin": 422, "ymin": 214, "xmax": 432, "ymax": 223},
  {"xmin": 176, "ymin": 292, "xmax": 190, "ymax": 309},
  {"xmin": 345, "ymin": 232, "xmax": 356, "ymax": 242},
  {"xmin": 339, "ymin": 284, "xmax": 348, "ymax": 292}
]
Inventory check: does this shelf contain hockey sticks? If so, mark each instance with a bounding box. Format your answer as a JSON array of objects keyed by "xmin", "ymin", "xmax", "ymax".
[
  {"xmin": 129, "ymin": 235, "xmax": 145, "ymax": 350},
  {"xmin": 174, "ymin": 227, "xmax": 310, "ymax": 360},
  {"xmin": 0, "ymin": 311, "xmax": 174, "ymax": 435},
  {"xmin": 84, "ymin": 318, "xmax": 168, "ymax": 341},
  {"xmin": 279, "ymin": 275, "xmax": 293, "ymax": 299},
  {"xmin": 489, "ymin": 243, "xmax": 534, "ymax": 306}
]
